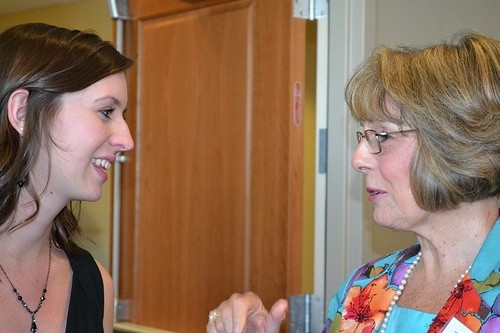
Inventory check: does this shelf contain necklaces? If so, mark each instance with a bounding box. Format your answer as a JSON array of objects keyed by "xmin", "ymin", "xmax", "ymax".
[
  {"xmin": 1, "ymin": 231, "xmax": 60, "ymax": 332},
  {"xmin": 375, "ymin": 251, "xmax": 473, "ymax": 332}
]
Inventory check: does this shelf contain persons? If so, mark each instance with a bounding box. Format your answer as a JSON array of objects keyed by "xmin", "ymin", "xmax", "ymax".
[
  {"xmin": 201, "ymin": 30, "xmax": 500, "ymax": 333},
  {"xmin": 0, "ymin": 21, "xmax": 137, "ymax": 333}
]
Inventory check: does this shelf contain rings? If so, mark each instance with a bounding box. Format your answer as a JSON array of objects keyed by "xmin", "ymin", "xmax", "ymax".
[{"xmin": 205, "ymin": 308, "xmax": 227, "ymax": 322}]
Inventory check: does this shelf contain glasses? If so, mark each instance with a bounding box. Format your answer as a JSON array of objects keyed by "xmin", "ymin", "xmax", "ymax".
[{"xmin": 357, "ymin": 129, "xmax": 418, "ymax": 154}]
